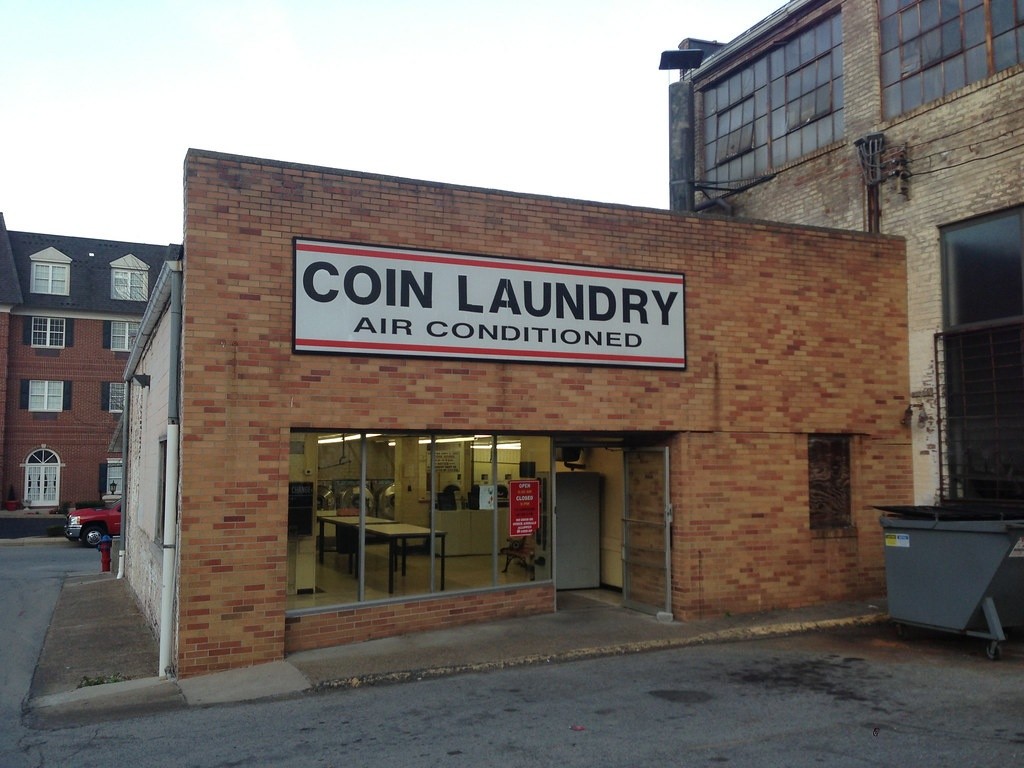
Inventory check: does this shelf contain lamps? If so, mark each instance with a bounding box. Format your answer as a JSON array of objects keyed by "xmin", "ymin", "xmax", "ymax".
[
  {"xmin": 110, "ymin": 480, "xmax": 118, "ymax": 495},
  {"xmin": 901, "ymin": 403, "xmax": 927, "ymax": 425},
  {"xmin": 132, "ymin": 375, "xmax": 151, "ymax": 389}
]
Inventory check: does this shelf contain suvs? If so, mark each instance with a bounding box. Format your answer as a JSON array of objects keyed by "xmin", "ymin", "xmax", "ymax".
[{"xmin": 63, "ymin": 499, "xmax": 121, "ymax": 548}]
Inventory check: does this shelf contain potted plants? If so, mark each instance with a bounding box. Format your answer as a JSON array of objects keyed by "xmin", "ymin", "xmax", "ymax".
[
  {"xmin": 7, "ymin": 486, "xmax": 18, "ymax": 511},
  {"xmin": 21, "ymin": 500, "xmax": 31, "ymax": 510}
]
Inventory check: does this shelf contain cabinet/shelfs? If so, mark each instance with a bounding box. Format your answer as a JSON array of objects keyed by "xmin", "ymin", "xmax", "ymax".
[{"xmin": 435, "ymin": 507, "xmax": 508, "ymax": 556}]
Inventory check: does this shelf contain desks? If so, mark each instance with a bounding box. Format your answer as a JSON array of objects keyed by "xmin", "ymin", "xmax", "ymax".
[
  {"xmin": 317, "ymin": 511, "xmax": 337, "ymax": 517},
  {"xmin": 319, "ymin": 516, "xmax": 399, "ymax": 575},
  {"xmin": 356, "ymin": 523, "xmax": 447, "ymax": 594}
]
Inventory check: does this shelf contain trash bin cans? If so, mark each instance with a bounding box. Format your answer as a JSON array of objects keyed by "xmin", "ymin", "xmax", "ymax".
[{"xmin": 870, "ymin": 500, "xmax": 1024, "ymax": 662}]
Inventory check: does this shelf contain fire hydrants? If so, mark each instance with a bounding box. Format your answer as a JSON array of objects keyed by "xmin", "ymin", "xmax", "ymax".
[{"xmin": 97, "ymin": 535, "xmax": 113, "ymax": 572}]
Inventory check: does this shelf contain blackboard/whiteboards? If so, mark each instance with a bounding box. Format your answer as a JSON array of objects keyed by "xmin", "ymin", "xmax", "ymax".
[{"xmin": 287, "ymin": 481, "xmax": 313, "ymax": 538}]
